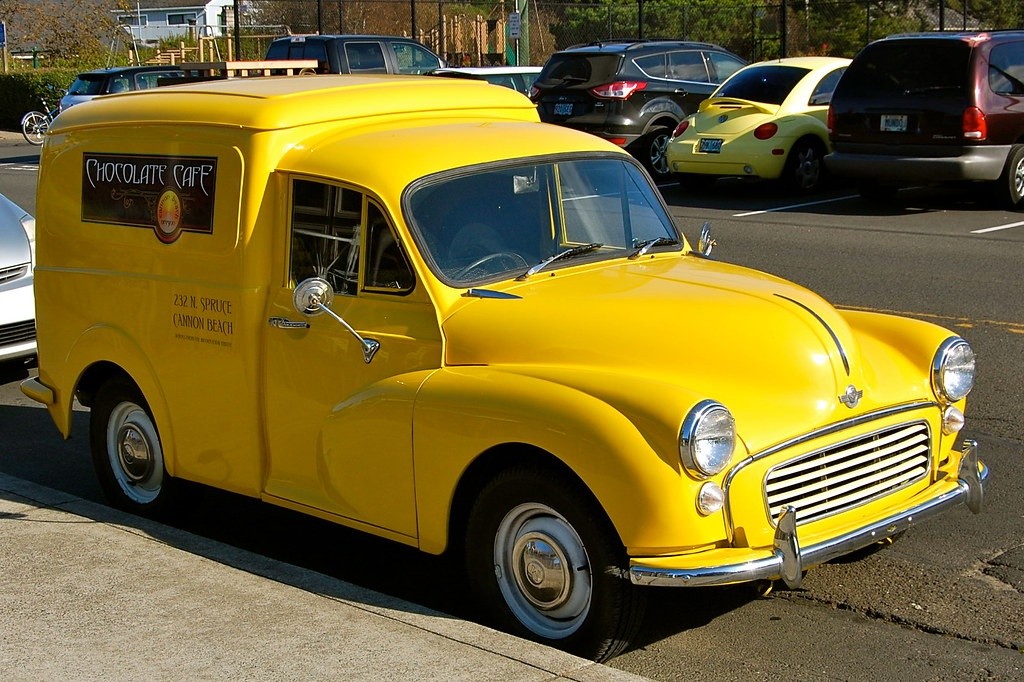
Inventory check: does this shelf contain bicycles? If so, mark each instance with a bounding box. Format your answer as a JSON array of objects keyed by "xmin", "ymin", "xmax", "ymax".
[{"xmin": 20, "ymin": 86, "xmax": 66, "ymax": 146}]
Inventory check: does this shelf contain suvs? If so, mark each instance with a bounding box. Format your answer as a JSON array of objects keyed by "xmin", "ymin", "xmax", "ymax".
[
  {"xmin": 526, "ymin": 38, "xmax": 753, "ymax": 185},
  {"xmin": 822, "ymin": 27, "xmax": 1024, "ymax": 213}
]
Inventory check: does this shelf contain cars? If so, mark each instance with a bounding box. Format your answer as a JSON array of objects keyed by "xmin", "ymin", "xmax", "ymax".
[
  {"xmin": 18, "ymin": 76, "xmax": 991, "ymax": 661},
  {"xmin": 665, "ymin": 56, "xmax": 855, "ymax": 202},
  {"xmin": 262, "ymin": 32, "xmax": 451, "ymax": 76},
  {"xmin": 0, "ymin": 194, "xmax": 37, "ymax": 369},
  {"xmin": 427, "ymin": 67, "xmax": 545, "ymax": 95},
  {"xmin": 60, "ymin": 65, "xmax": 199, "ymax": 113}
]
